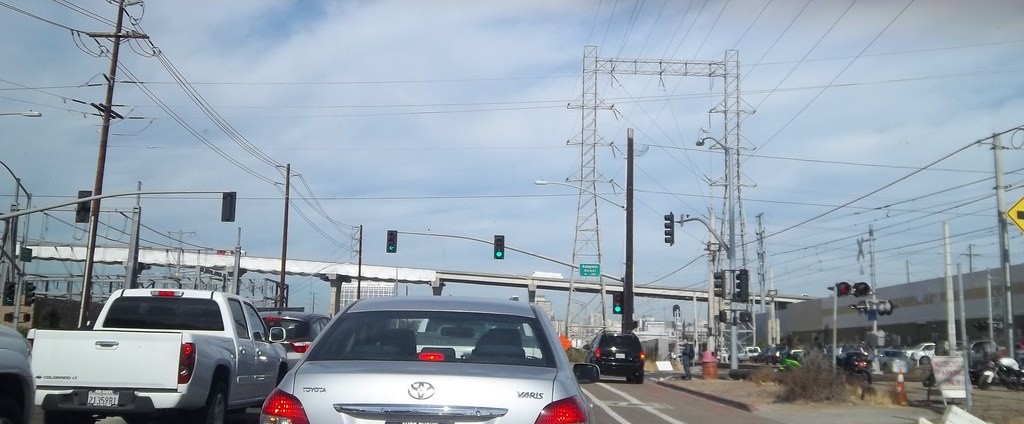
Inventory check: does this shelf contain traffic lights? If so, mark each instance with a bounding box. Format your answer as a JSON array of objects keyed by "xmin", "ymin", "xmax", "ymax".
[
  {"xmin": 494, "ymin": 235, "xmax": 504, "ymax": 259},
  {"xmin": 664, "ymin": 214, "xmax": 674, "ymax": 244},
  {"xmin": 854, "ymin": 282, "xmax": 870, "ymax": 297},
  {"xmin": 836, "ymin": 282, "xmax": 851, "ymax": 298},
  {"xmin": 386, "ymin": 230, "xmax": 397, "ymax": 253},
  {"xmin": 613, "ymin": 292, "xmax": 623, "ymax": 314},
  {"xmin": 736, "ymin": 269, "xmax": 749, "ymax": 303},
  {"xmin": 714, "ymin": 271, "xmax": 726, "ymax": 299}
]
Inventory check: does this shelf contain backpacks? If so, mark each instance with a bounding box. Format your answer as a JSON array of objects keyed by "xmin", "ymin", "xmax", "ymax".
[{"xmin": 684, "ymin": 343, "xmax": 695, "ymax": 360}]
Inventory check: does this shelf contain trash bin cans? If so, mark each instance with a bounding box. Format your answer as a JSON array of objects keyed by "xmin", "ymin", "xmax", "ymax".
[{"xmin": 701, "ymin": 351, "xmax": 718, "ymax": 380}]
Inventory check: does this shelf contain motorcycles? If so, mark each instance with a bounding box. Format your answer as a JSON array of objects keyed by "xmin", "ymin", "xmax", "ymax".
[{"xmin": 979, "ymin": 357, "xmax": 1024, "ymax": 390}]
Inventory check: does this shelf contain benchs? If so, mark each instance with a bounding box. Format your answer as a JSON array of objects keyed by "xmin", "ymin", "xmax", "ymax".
[{"xmin": 352, "ymin": 345, "xmax": 523, "ymax": 364}]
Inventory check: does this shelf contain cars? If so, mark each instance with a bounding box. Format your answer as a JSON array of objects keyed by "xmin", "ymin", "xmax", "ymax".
[
  {"xmin": 261, "ymin": 296, "xmax": 601, "ymax": 424},
  {"xmin": 902, "ymin": 343, "xmax": 936, "ymax": 364},
  {"xmin": 257, "ymin": 312, "xmax": 331, "ymax": 372},
  {"xmin": 738, "ymin": 346, "xmax": 866, "ymax": 363},
  {"xmin": 0, "ymin": 325, "xmax": 36, "ymax": 424},
  {"xmin": 869, "ymin": 348, "xmax": 916, "ymax": 375},
  {"xmin": 837, "ymin": 351, "xmax": 871, "ymax": 374},
  {"xmin": 956, "ymin": 340, "xmax": 1006, "ymax": 365}
]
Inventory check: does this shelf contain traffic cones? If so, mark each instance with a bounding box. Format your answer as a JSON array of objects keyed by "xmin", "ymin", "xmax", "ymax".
[{"xmin": 896, "ymin": 371, "xmax": 911, "ymax": 406}]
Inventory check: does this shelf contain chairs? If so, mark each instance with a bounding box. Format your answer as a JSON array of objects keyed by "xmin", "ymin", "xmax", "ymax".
[
  {"xmin": 369, "ymin": 329, "xmax": 416, "ymax": 355},
  {"xmin": 480, "ymin": 329, "xmax": 522, "ymax": 345}
]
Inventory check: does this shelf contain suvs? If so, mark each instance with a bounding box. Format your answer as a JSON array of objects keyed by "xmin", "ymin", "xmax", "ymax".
[{"xmin": 583, "ymin": 330, "xmax": 643, "ymax": 384}]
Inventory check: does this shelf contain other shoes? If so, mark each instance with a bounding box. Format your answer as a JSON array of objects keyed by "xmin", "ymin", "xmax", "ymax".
[{"xmin": 685, "ymin": 376, "xmax": 691, "ymax": 380}]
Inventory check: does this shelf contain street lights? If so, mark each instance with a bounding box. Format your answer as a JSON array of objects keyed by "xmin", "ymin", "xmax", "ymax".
[
  {"xmin": 696, "ymin": 137, "xmax": 739, "ymax": 371},
  {"xmin": 535, "ymin": 181, "xmax": 634, "ymax": 335}
]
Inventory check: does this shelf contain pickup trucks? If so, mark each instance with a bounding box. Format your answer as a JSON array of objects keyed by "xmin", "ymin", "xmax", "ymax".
[{"xmin": 26, "ymin": 288, "xmax": 287, "ymax": 424}]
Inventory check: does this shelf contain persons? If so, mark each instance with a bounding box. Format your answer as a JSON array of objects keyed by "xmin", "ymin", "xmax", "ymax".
[{"xmin": 682, "ymin": 337, "xmax": 691, "ymax": 380}]
